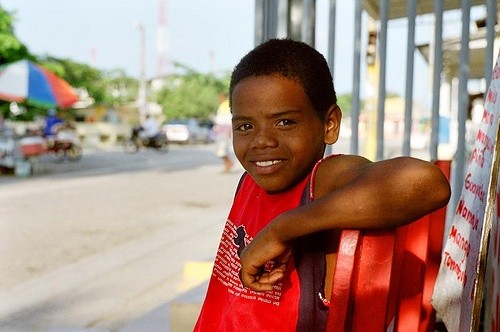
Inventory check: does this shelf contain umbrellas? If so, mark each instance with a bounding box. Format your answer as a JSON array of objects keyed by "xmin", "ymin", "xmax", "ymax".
[{"xmin": 0, "ymin": 58, "xmax": 80, "ymax": 111}]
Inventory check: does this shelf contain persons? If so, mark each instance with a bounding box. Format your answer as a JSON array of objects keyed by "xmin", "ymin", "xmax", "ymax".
[
  {"xmin": 192, "ymin": 38, "xmax": 452, "ymax": 332},
  {"xmin": 0, "ymin": 92, "xmax": 237, "ymax": 173}
]
